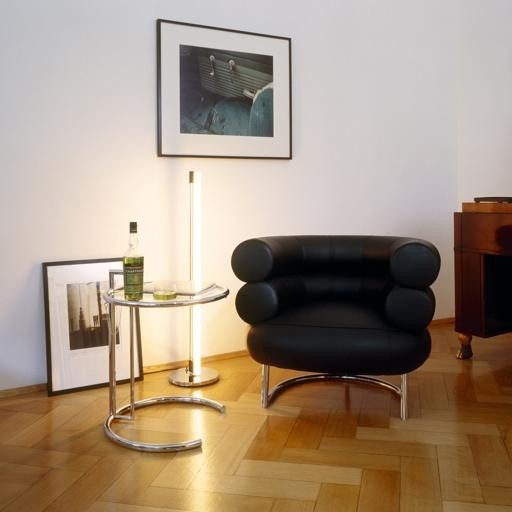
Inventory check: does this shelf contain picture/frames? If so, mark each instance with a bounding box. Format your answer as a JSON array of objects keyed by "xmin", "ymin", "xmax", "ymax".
[
  {"xmin": 156, "ymin": 20, "xmax": 293, "ymax": 162},
  {"xmin": 42, "ymin": 255, "xmax": 152, "ymax": 398}
]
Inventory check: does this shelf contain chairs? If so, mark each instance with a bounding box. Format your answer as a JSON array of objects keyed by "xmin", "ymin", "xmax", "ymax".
[{"xmin": 231, "ymin": 234, "xmax": 442, "ymax": 422}]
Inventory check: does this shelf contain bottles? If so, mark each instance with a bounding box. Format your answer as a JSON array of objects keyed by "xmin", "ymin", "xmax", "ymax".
[{"xmin": 123, "ymin": 221, "xmax": 144, "ymax": 301}]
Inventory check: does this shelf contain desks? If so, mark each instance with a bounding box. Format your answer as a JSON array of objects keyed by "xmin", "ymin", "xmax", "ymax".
[
  {"xmin": 99, "ymin": 281, "xmax": 230, "ymax": 455},
  {"xmin": 452, "ymin": 209, "xmax": 511, "ymax": 359}
]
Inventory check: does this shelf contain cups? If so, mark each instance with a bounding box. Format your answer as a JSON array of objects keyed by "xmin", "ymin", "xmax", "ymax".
[{"xmin": 152, "ymin": 281, "xmax": 176, "ymax": 301}]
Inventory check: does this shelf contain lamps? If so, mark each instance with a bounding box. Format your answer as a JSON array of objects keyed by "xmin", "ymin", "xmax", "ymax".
[{"xmin": 168, "ymin": 169, "xmax": 223, "ymax": 388}]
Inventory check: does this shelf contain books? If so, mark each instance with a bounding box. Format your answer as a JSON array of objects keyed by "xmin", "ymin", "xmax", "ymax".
[{"xmin": 474, "ymin": 197, "xmax": 512, "ymax": 204}]
